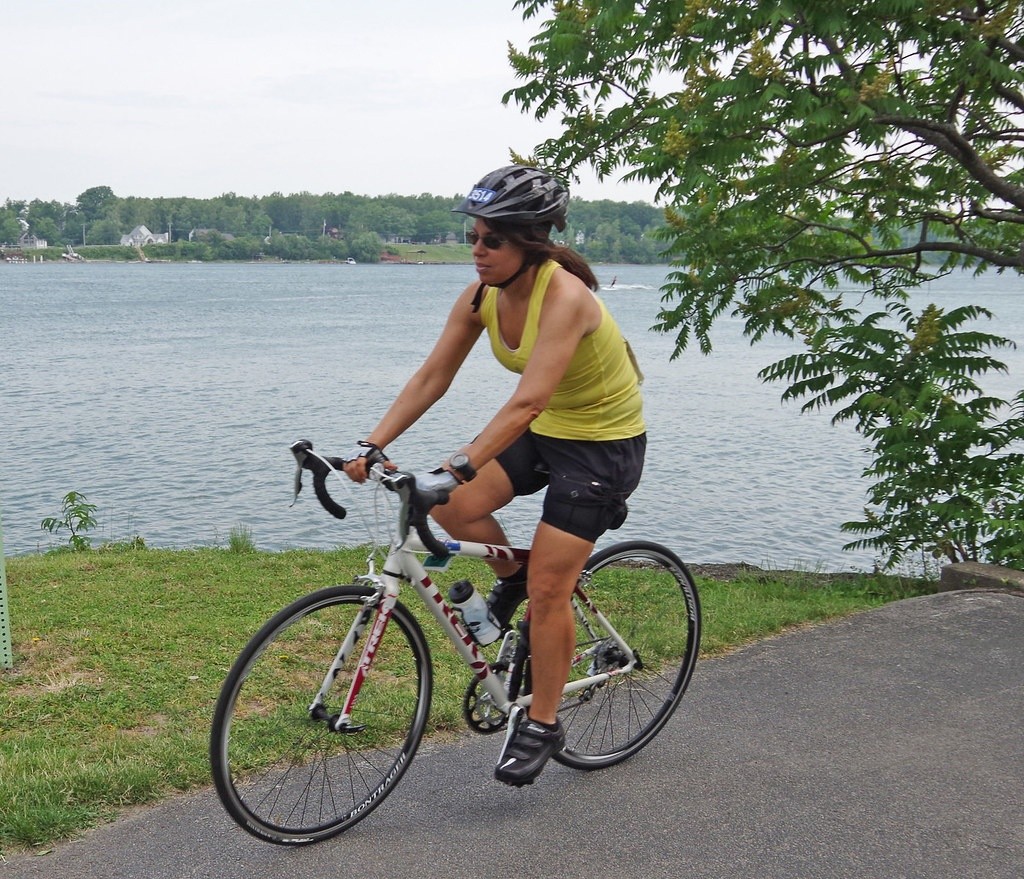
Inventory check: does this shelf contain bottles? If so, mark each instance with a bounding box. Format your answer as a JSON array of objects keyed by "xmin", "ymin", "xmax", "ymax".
[{"xmin": 450, "ymin": 579, "xmax": 501, "ymax": 645}]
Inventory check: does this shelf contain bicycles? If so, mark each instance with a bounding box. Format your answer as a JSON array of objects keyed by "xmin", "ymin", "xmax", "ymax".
[{"xmin": 211, "ymin": 439, "xmax": 701, "ymax": 846}]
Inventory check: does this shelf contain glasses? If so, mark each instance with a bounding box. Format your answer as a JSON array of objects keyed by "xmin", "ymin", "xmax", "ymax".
[{"xmin": 464, "ymin": 231, "xmax": 513, "ymax": 250}]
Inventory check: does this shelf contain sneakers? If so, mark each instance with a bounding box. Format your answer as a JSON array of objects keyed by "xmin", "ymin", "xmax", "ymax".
[
  {"xmin": 496, "ymin": 718, "xmax": 566, "ymax": 789},
  {"xmin": 487, "ymin": 571, "xmax": 529, "ymax": 630}
]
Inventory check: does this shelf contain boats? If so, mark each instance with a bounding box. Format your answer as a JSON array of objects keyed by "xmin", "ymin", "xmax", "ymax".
[{"xmin": 343, "ymin": 257, "xmax": 356, "ymax": 265}]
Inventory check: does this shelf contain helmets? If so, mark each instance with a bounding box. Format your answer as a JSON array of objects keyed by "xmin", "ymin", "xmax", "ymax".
[{"xmin": 451, "ymin": 164, "xmax": 569, "ymax": 233}]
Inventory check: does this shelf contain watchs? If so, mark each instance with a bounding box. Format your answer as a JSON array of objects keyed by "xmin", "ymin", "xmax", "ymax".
[{"xmin": 448, "ymin": 453, "xmax": 477, "ymax": 483}]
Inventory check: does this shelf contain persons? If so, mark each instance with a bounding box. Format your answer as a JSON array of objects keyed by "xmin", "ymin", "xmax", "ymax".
[
  {"xmin": 610, "ymin": 276, "xmax": 617, "ymax": 288},
  {"xmin": 343, "ymin": 166, "xmax": 648, "ymax": 782}
]
